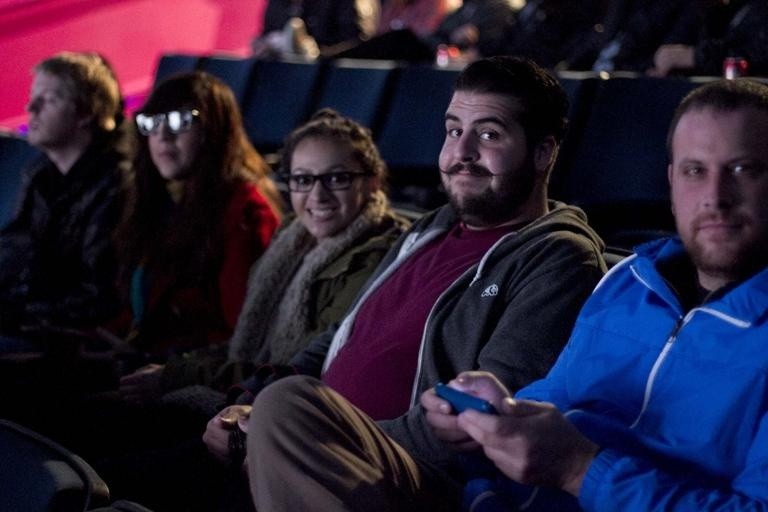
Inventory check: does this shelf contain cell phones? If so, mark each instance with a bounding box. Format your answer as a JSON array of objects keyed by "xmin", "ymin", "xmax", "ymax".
[{"xmin": 435, "ymin": 384, "xmax": 502, "ymax": 415}]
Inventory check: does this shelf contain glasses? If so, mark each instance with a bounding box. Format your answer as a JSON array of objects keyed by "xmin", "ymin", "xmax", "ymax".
[
  {"xmin": 135, "ymin": 107, "xmax": 200, "ymax": 136},
  {"xmin": 281, "ymin": 171, "xmax": 371, "ymax": 192}
]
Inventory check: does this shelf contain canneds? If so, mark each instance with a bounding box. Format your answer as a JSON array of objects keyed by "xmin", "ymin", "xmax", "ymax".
[
  {"xmin": 724, "ymin": 57, "xmax": 748, "ymax": 80},
  {"xmin": 436, "ymin": 45, "xmax": 458, "ymax": 66}
]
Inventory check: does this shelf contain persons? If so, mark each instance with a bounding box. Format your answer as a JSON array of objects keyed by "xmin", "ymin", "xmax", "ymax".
[
  {"xmin": 119, "ymin": 119, "xmax": 407, "ymax": 462},
  {"xmin": 251, "ymin": 0, "xmax": 766, "ymax": 87},
  {"xmin": 420, "ymin": 72, "xmax": 767, "ymax": 509},
  {"xmin": 0, "ymin": 49, "xmax": 135, "ymax": 378},
  {"xmin": 201, "ymin": 56, "xmax": 605, "ymax": 510},
  {"xmin": 82, "ymin": 71, "xmax": 280, "ymax": 408}
]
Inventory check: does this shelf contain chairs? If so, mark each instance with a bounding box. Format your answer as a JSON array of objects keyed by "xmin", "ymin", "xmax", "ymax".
[
  {"xmin": 141, "ymin": 54, "xmax": 767, "ymax": 251},
  {"xmin": 0, "ymin": 135, "xmax": 41, "ymax": 227},
  {"xmin": 88, "ymin": 499, "xmax": 158, "ymax": 512},
  {"xmin": 1, "ymin": 418, "xmax": 110, "ymax": 512}
]
